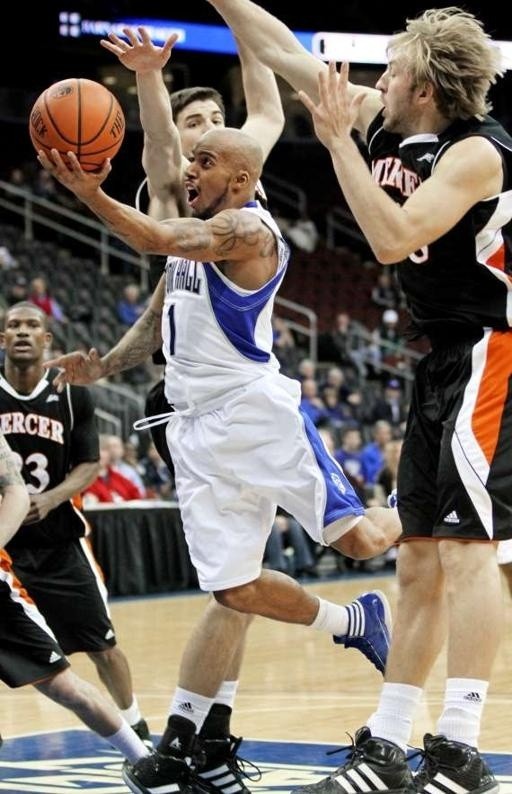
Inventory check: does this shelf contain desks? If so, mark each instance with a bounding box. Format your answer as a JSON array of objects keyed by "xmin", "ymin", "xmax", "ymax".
[{"xmin": 83, "ymin": 498, "xmax": 198, "ymax": 596}]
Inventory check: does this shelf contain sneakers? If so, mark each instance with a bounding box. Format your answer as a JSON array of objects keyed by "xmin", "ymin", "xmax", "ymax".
[
  {"xmin": 332, "ymin": 588, "xmax": 395, "ymax": 678},
  {"xmin": 124, "ymin": 723, "xmax": 264, "ymax": 794},
  {"xmin": 286, "ymin": 725, "xmax": 419, "ymax": 792},
  {"xmin": 401, "ymin": 733, "xmax": 507, "ymax": 794}
]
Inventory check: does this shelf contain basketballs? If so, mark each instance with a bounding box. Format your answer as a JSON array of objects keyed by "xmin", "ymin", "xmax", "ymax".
[{"xmin": 31, "ymin": 79, "xmax": 125, "ymax": 173}]
